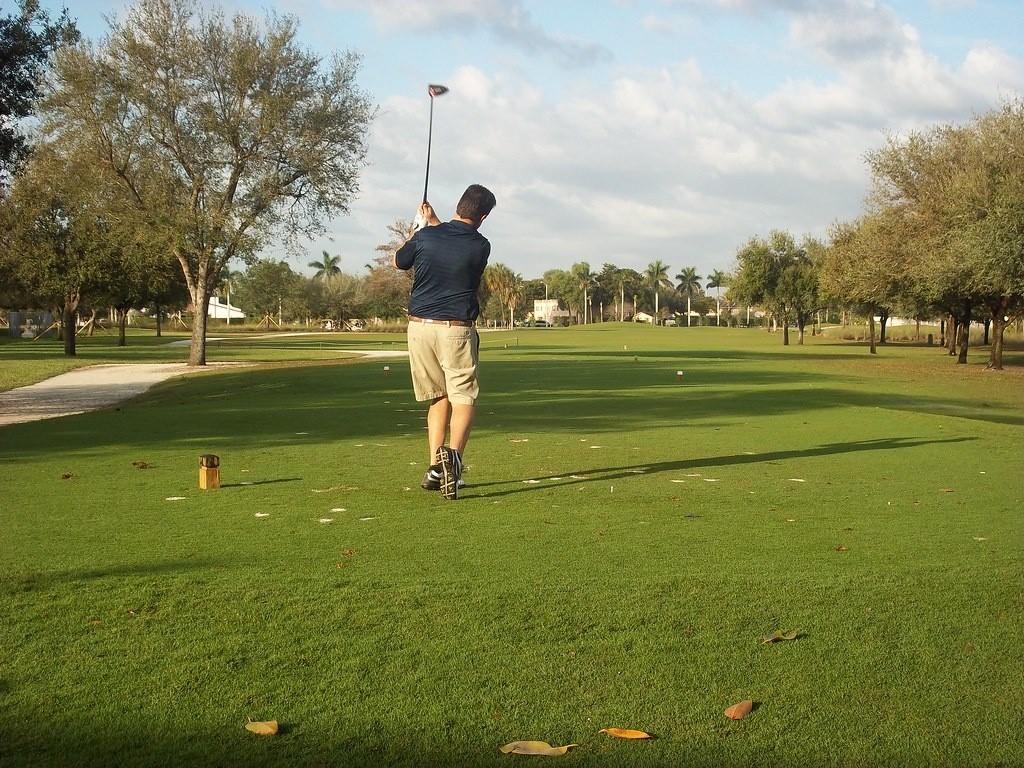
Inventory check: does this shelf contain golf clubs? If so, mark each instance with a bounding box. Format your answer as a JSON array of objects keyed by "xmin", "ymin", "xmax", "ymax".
[{"xmin": 423, "ymin": 84, "xmax": 449, "ymax": 204}]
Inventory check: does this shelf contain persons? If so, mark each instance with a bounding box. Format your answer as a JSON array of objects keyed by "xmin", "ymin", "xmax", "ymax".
[{"xmin": 392, "ymin": 184, "xmax": 498, "ymax": 501}]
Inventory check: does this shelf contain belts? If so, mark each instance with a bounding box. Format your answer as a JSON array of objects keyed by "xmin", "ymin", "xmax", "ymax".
[{"xmin": 409, "ymin": 316, "xmax": 472, "ymax": 327}]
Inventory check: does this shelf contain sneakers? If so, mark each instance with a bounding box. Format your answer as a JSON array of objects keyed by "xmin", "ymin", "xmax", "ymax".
[
  {"xmin": 421, "ymin": 469, "xmax": 466, "ymax": 490},
  {"xmin": 436, "ymin": 446, "xmax": 462, "ymax": 500}
]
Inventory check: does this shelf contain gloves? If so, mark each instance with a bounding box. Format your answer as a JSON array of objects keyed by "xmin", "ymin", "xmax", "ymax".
[{"xmin": 413, "ymin": 213, "xmax": 428, "ymax": 232}]
